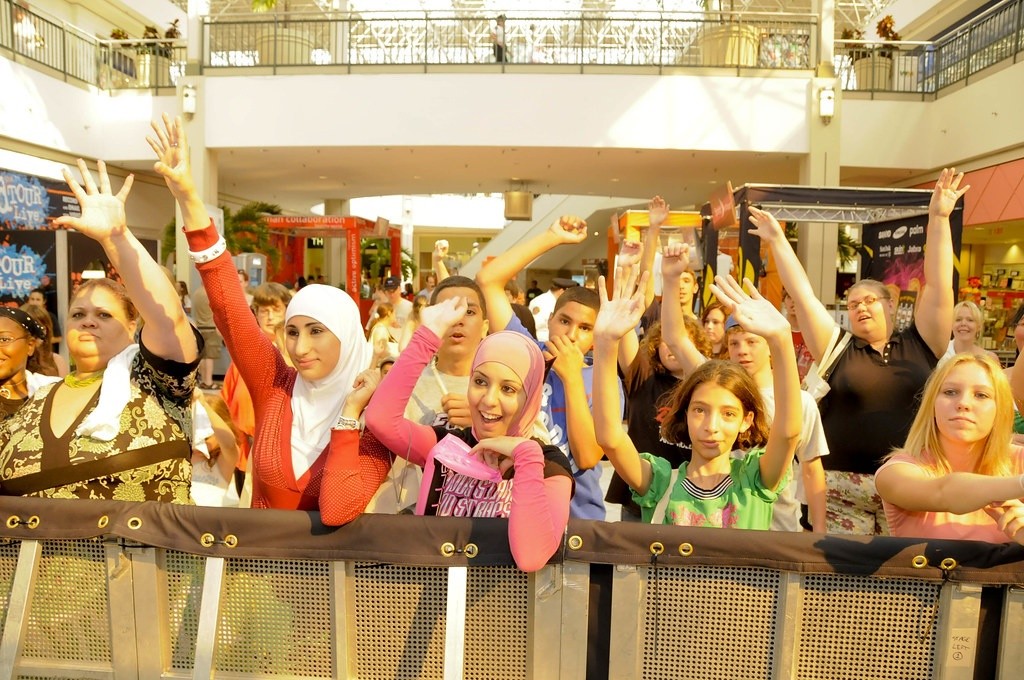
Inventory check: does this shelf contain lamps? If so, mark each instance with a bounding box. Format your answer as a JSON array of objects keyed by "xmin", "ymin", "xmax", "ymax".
[
  {"xmin": 502, "ymin": 175, "xmax": 536, "ymax": 225},
  {"xmin": 818, "ymin": 84, "xmax": 837, "ymax": 126},
  {"xmin": 179, "ymin": 85, "xmax": 198, "ymax": 122}
]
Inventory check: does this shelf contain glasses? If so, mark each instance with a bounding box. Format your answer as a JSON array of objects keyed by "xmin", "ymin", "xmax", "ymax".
[
  {"xmin": 846, "ymin": 295, "xmax": 890, "ymax": 310},
  {"xmin": 0, "ymin": 333, "xmax": 31, "ymax": 347}
]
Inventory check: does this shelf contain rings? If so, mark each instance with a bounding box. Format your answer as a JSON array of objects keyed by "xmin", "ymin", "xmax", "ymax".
[
  {"xmin": 174, "ymin": 143, "xmax": 177, "ymax": 146},
  {"xmin": 170, "ymin": 143, "xmax": 174, "ymax": 147}
]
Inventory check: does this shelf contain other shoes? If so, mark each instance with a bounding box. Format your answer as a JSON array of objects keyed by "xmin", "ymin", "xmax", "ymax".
[{"xmin": 200, "ymin": 382, "xmax": 223, "ymax": 390}]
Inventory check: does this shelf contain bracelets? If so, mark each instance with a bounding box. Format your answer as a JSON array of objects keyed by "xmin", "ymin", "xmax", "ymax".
[
  {"xmin": 337, "ymin": 417, "xmax": 357, "ymax": 427},
  {"xmin": 187, "ymin": 232, "xmax": 226, "ymax": 264}
]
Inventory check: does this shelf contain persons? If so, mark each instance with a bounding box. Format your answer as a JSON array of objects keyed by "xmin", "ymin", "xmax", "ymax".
[
  {"xmin": 0, "ymin": 113, "xmax": 1024, "ymax": 573},
  {"xmin": 491, "ymin": 14, "xmax": 508, "ymax": 62}
]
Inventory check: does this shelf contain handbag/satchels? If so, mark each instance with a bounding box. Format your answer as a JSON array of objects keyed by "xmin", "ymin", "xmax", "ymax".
[{"xmin": 792, "ymin": 324, "xmax": 852, "ymax": 405}]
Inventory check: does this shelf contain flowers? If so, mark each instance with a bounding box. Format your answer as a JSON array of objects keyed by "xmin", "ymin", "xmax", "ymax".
[
  {"xmin": 110, "ymin": 20, "xmax": 189, "ymax": 56},
  {"xmin": 841, "ymin": 15, "xmax": 898, "ymax": 59}
]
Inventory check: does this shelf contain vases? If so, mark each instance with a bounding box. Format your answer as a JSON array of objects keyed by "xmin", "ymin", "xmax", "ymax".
[
  {"xmin": 852, "ymin": 62, "xmax": 892, "ymax": 89},
  {"xmin": 135, "ymin": 59, "xmax": 172, "ymax": 88}
]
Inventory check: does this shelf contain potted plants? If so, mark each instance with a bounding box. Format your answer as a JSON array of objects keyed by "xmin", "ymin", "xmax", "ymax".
[
  {"xmin": 258, "ymin": 2, "xmax": 315, "ymax": 64},
  {"xmin": 699, "ymin": 2, "xmax": 763, "ymax": 69}
]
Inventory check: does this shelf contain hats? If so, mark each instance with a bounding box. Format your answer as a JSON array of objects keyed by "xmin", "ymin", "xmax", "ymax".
[
  {"xmin": 552, "ymin": 278, "xmax": 579, "ymax": 288},
  {"xmin": 384, "ymin": 277, "xmax": 400, "ymax": 289},
  {"xmin": 725, "ymin": 313, "xmax": 739, "ymax": 332}
]
